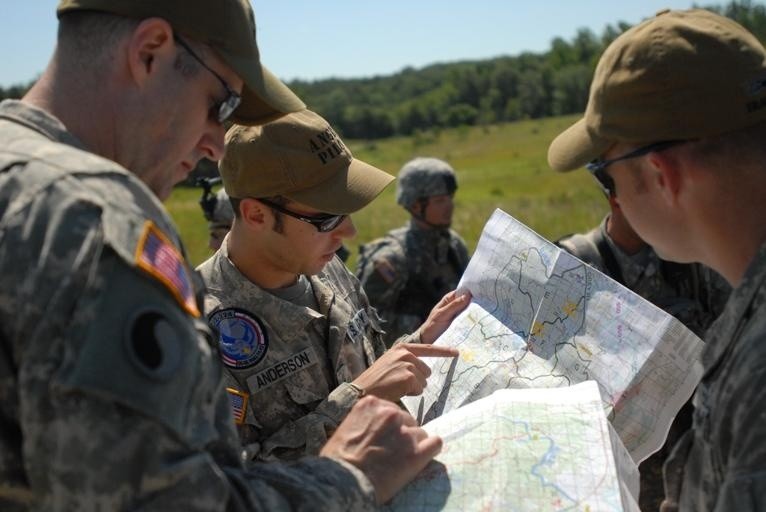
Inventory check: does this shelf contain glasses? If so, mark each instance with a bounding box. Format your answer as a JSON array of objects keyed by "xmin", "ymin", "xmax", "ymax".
[
  {"xmin": 587, "ymin": 140, "xmax": 690, "ymax": 195},
  {"xmin": 170, "ymin": 31, "xmax": 242, "ymax": 123},
  {"xmin": 245, "ymin": 195, "xmax": 348, "ymax": 233}
]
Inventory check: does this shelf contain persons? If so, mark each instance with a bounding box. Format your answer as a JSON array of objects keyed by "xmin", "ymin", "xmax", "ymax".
[
  {"xmin": 547, "ymin": 9, "xmax": 765, "ymax": 512},
  {"xmin": 0, "ymin": 0, "xmax": 443, "ymax": 512},
  {"xmin": 193, "ymin": 107, "xmax": 472, "ymax": 463}
]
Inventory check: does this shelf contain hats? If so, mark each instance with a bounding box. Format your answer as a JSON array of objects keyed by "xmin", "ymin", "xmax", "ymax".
[
  {"xmin": 395, "ymin": 156, "xmax": 457, "ymax": 208},
  {"xmin": 55, "ymin": 0, "xmax": 307, "ymax": 125},
  {"xmin": 218, "ymin": 110, "xmax": 398, "ymax": 212},
  {"xmin": 546, "ymin": 8, "xmax": 766, "ymax": 174}
]
